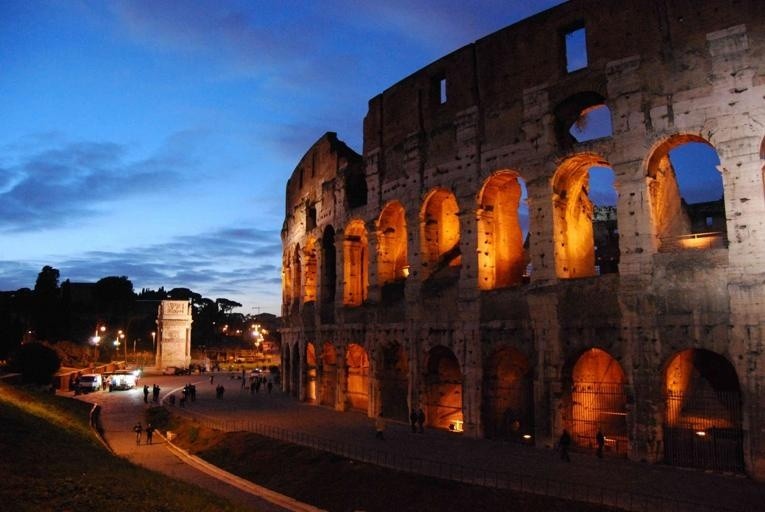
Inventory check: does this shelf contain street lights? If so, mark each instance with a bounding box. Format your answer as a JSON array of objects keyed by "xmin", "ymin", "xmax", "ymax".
[
  {"xmin": 151, "ymin": 332, "xmax": 155, "ymax": 365},
  {"xmin": 95, "ymin": 324, "xmax": 106, "ymax": 362},
  {"xmin": 116, "ymin": 329, "xmax": 125, "ymax": 362}
]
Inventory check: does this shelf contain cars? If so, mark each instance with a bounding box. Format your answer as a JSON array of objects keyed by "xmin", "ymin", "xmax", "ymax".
[
  {"xmin": 76, "ymin": 373, "xmax": 102, "ymax": 392},
  {"xmin": 106, "ymin": 369, "xmax": 137, "ymax": 391}
]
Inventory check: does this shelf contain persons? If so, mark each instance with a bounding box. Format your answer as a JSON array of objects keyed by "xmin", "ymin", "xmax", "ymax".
[
  {"xmin": 410, "ymin": 409, "xmax": 417, "ymax": 433},
  {"xmin": 560, "ymin": 429, "xmax": 570, "ymax": 462},
  {"xmin": 146, "ymin": 423, "xmax": 154, "ymax": 445},
  {"xmin": 144, "ymin": 368, "xmax": 272, "ymax": 408},
  {"xmin": 596, "ymin": 428, "xmax": 604, "ymax": 458},
  {"xmin": 134, "ymin": 422, "xmax": 143, "ymax": 447},
  {"xmin": 376, "ymin": 413, "xmax": 386, "ymax": 440},
  {"xmin": 418, "ymin": 409, "xmax": 425, "ymax": 433}
]
